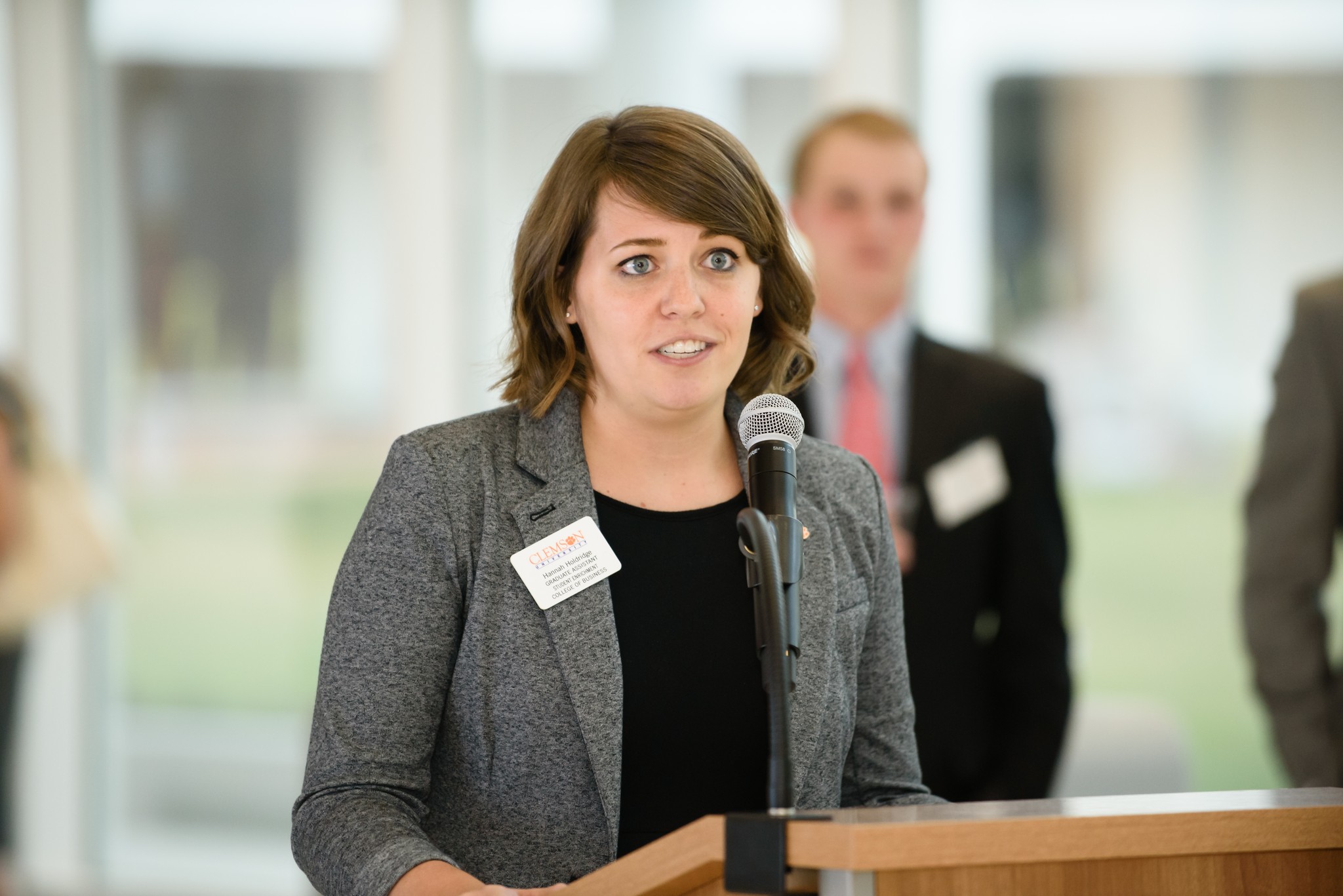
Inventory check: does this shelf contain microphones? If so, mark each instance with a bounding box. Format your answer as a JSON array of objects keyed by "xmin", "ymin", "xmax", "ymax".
[{"xmin": 738, "ymin": 393, "xmax": 805, "ymax": 689}]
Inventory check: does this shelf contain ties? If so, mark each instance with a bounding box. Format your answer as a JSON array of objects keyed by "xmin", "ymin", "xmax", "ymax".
[{"xmin": 840, "ymin": 354, "xmax": 892, "ymax": 492}]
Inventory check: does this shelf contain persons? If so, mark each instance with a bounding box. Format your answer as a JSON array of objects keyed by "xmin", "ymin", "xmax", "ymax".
[
  {"xmin": 1, "ymin": 364, "xmax": 102, "ymax": 879},
  {"xmin": 291, "ymin": 104, "xmax": 954, "ymax": 896},
  {"xmin": 1237, "ymin": 270, "xmax": 1342, "ymax": 789},
  {"xmin": 786, "ymin": 111, "xmax": 1074, "ymax": 803}
]
